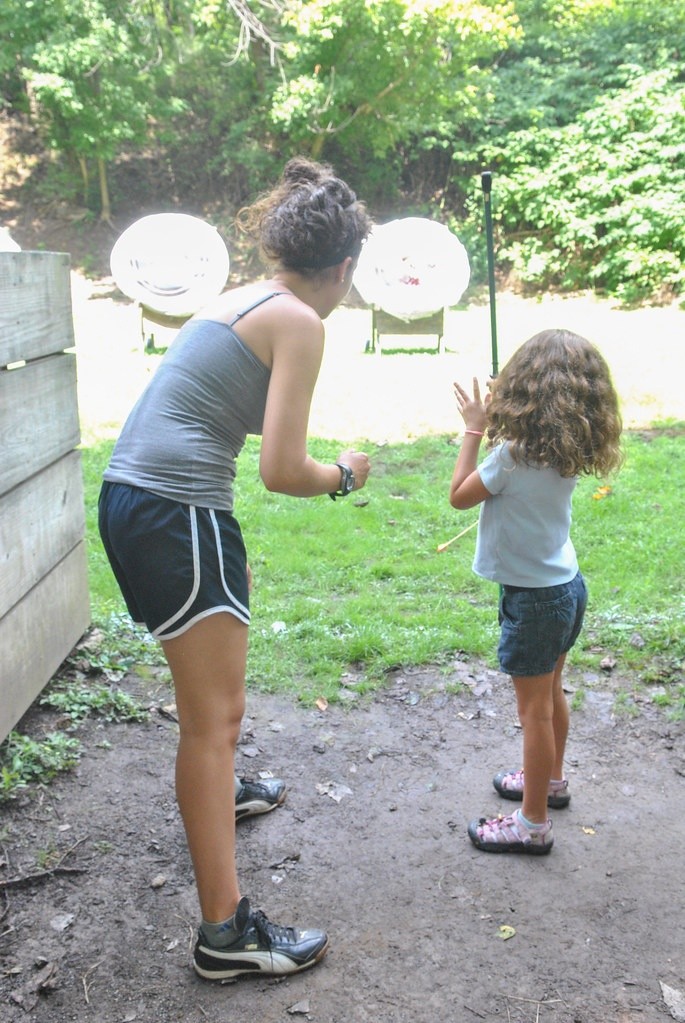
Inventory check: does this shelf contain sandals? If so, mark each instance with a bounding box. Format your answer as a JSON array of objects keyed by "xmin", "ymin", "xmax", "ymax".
[
  {"xmin": 469, "ymin": 807, "xmax": 555, "ymax": 856},
  {"xmin": 492, "ymin": 768, "xmax": 571, "ymax": 811}
]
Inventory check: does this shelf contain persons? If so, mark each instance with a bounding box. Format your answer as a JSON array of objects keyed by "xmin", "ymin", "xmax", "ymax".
[
  {"xmin": 97, "ymin": 154, "xmax": 374, "ymax": 984},
  {"xmin": 449, "ymin": 329, "xmax": 623, "ymax": 859}
]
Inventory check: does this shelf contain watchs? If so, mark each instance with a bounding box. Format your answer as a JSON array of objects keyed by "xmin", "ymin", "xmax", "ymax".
[{"xmin": 327, "ymin": 463, "xmax": 356, "ymax": 502}]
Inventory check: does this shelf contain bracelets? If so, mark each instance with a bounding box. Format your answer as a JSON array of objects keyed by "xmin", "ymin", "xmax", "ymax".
[{"xmin": 464, "ymin": 430, "xmax": 485, "ymax": 436}]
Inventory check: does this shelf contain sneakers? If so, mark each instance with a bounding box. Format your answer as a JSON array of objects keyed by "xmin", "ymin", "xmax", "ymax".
[
  {"xmin": 235, "ymin": 776, "xmax": 288, "ymax": 820},
  {"xmin": 193, "ymin": 894, "xmax": 331, "ymax": 980}
]
